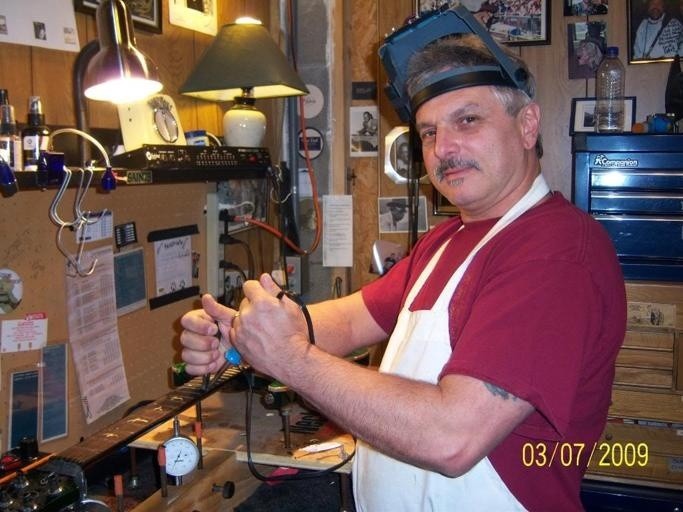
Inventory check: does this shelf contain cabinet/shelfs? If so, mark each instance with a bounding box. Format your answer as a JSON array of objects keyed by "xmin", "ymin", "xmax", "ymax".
[{"xmin": 585, "ymin": 284, "xmax": 683, "ymax": 494}]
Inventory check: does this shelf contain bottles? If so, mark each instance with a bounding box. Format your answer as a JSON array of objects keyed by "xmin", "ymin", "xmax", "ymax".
[
  {"xmin": 0, "ymin": 89, "xmax": 22, "ymax": 174},
  {"xmin": 595, "ymin": 47, "xmax": 624, "ymax": 133},
  {"xmin": 20, "ymin": 96, "xmax": 51, "ymax": 171}
]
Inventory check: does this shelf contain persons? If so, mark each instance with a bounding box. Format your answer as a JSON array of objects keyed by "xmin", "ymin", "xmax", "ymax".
[
  {"xmin": 359, "ymin": 111, "xmax": 377, "ymax": 135},
  {"xmin": 632, "ymin": 1, "xmax": 683, "ymax": 60},
  {"xmin": 567, "ymin": 1, "xmax": 607, "ymax": 15},
  {"xmin": 378, "ymin": 197, "xmax": 419, "ymax": 233},
  {"xmin": 469, "ymin": 1, "xmax": 541, "ymax": 41},
  {"xmin": 178, "ymin": 33, "xmax": 627, "ymax": 511},
  {"xmin": 575, "ymin": 23, "xmax": 605, "ymax": 64},
  {"xmin": 395, "ymin": 142, "xmax": 415, "ymax": 179}
]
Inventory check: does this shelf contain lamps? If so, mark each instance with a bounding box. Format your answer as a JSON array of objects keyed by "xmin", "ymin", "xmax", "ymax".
[
  {"xmin": 179, "ymin": 21, "xmax": 308, "ymax": 147},
  {"xmin": 68, "ymin": 1, "xmax": 166, "ymax": 165}
]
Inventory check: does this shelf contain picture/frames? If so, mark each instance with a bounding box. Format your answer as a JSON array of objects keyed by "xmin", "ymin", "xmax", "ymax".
[
  {"xmin": 412, "ymin": 0, "xmax": 553, "ymax": 47},
  {"xmin": 625, "ymin": 1, "xmax": 681, "ymax": 65},
  {"xmin": 430, "ymin": 186, "xmax": 462, "ymax": 218},
  {"xmin": 567, "ymin": 94, "xmax": 637, "ymax": 137},
  {"xmin": 382, "ymin": 124, "xmax": 430, "ymax": 186},
  {"xmin": 73, "ymin": 0, "xmax": 165, "ymax": 37}
]
